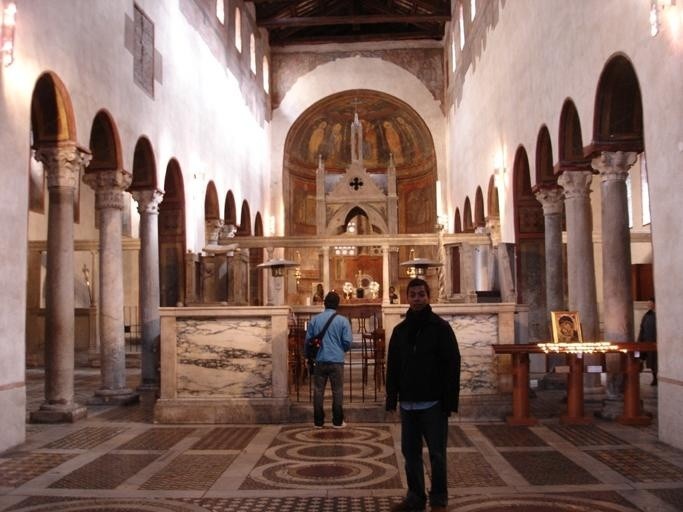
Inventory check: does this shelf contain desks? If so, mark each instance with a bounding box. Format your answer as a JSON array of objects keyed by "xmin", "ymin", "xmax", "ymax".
[{"xmin": 492, "ymin": 343, "xmax": 656, "ymax": 425}]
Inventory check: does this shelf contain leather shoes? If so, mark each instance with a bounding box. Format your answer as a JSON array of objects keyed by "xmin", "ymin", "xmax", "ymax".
[{"xmin": 391, "ymin": 501, "xmax": 447, "ymax": 512}]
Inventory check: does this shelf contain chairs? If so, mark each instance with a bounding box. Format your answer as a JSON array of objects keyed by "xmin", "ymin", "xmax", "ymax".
[{"xmin": 288, "ymin": 311, "xmax": 385, "ymax": 402}]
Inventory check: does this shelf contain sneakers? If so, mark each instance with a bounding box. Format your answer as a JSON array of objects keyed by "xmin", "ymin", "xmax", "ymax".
[
  {"xmin": 332, "ymin": 421, "xmax": 346, "ymax": 428},
  {"xmin": 312, "ymin": 421, "xmax": 323, "ymax": 429}
]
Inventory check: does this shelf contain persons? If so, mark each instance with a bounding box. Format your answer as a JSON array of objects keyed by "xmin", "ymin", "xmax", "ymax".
[
  {"xmin": 298, "ymin": 114, "xmax": 423, "ymax": 170},
  {"xmin": 635, "ymin": 296, "xmax": 658, "ymax": 386},
  {"xmin": 384, "ymin": 278, "xmax": 461, "ymax": 512},
  {"xmin": 561, "ymin": 327, "xmax": 574, "ymax": 342},
  {"xmin": 303, "ymin": 291, "xmax": 352, "ymax": 429},
  {"xmin": 564, "ymin": 321, "xmax": 578, "ymax": 342},
  {"xmin": 389, "ymin": 286, "xmax": 398, "ymax": 304},
  {"xmin": 312, "ymin": 284, "xmax": 323, "ymax": 304}
]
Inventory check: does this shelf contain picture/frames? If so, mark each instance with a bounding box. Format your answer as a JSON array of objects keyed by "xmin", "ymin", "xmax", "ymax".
[
  {"xmin": 550, "ymin": 310, "xmax": 583, "ymax": 343},
  {"xmin": 311, "ymin": 283, "xmax": 323, "ymax": 305},
  {"xmin": 389, "ymin": 285, "xmax": 400, "ymax": 304}
]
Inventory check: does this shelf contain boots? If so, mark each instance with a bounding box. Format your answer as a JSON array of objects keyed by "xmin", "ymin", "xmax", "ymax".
[{"xmin": 651, "ymin": 373, "xmax": 657, "ymax": 385}]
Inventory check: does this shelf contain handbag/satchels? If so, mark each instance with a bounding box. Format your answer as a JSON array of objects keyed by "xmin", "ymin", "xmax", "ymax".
[{"xmin": 307, "ymin": 337, "xmax": 321, "ymax": 358}]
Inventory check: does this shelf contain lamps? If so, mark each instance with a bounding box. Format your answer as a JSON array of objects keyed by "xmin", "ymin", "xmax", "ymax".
[
  {"xmin": 257, "ymin": 247, "xmax": 300, "ymax": 305},
  {"xmin": 400, "ymin": 259, "xmax": 444, "ymax": 280}
]
